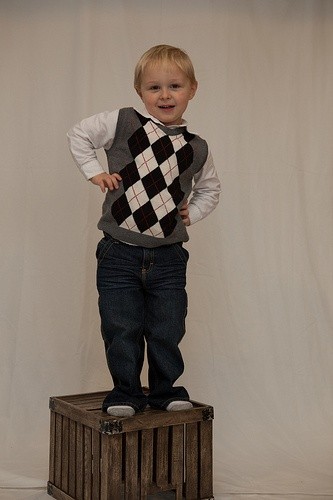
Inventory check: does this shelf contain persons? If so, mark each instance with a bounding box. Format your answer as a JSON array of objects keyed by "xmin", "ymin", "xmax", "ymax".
[{"xmin": 64, "ymin": 43, "xmax": 222, "ymax": 419}]
